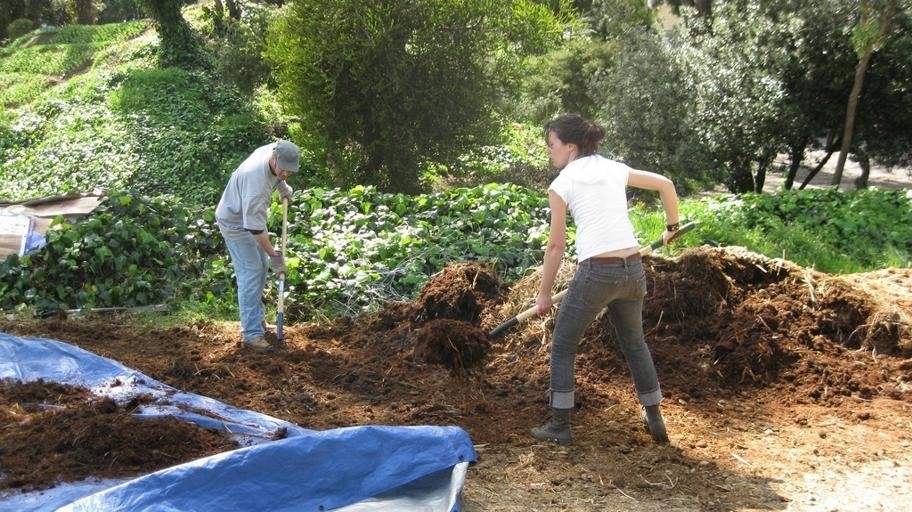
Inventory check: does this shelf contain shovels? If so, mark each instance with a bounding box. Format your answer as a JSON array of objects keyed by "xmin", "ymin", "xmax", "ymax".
[
  {"xmin": 404, "ymin": 219, "xmax": 703, "ymax": 370},
  {"xmin": 31, "ymin": 303, "xmax": 170, "ymax": 318}
]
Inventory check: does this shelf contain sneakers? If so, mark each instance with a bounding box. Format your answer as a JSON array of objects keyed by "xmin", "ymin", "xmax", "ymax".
[{"xmin": 244, "ymin": 335, "xmax": 274, "ymax": 351}]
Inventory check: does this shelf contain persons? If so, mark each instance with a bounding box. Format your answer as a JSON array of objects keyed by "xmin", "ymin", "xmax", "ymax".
[
  {"xmin": 528, "ymin": 114, "xmax": 680, "ymax": 442},
  {"xmin": 216, "ymin": 140, "xmax": 300, "ymax": 351}
]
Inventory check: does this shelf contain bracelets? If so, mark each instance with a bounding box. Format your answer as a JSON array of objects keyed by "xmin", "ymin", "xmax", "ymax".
[{"xmin": 665, "ymin": 222, "xmax": 679, "ymax": 232}]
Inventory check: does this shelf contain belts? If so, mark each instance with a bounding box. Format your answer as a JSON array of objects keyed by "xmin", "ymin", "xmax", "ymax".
[{"xmin": 580, "ymin": 252, "xmax": 642, "ymax": 269}]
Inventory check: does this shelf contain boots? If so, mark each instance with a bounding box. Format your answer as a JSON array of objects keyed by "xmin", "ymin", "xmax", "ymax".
[
  {"xmin": 640, "ymin": 404, "xmax": 668, "ymax": 442},
  {"xmin": 529, "ymin": 407, "xmax": 574, "ymax": 447}
]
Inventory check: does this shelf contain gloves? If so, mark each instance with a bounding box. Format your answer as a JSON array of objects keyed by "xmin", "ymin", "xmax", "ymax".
[
  {"xmin": 275, "ymin": 178, "xmax": 294, "ymax": 204},
  {"xmin": 269, "ymin": 250, "xmax": 289, "ymax": 279}
]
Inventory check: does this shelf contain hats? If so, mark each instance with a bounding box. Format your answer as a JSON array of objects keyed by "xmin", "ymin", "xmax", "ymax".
[{"xmin": 275, "ymin": 139, "xmax": 300, "ymax": 173}]
